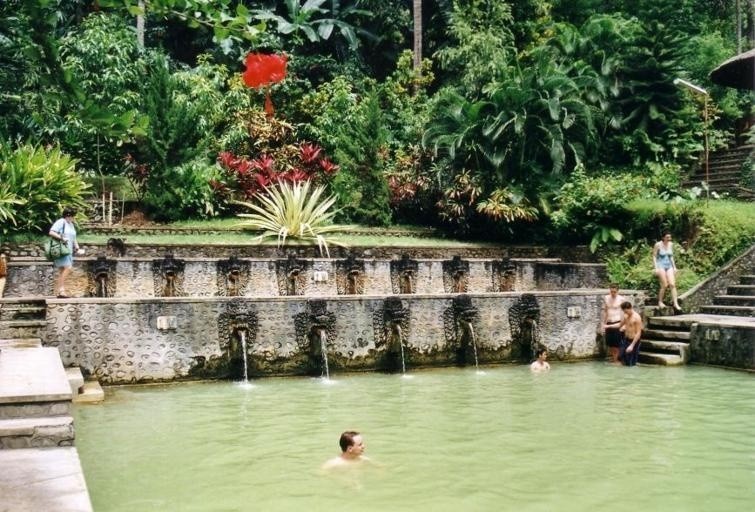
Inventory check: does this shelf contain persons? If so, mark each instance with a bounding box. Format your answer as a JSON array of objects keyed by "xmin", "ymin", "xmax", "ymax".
[
  {"xmin": 603, "ymin": 299, "xmax": 645, "ymax": 368},
  {"xmin": 599, "ymin": 282, "xmax": 628, "ymax": 368},
  {"xmin": 327, "ymin": 429, "xmax": 368, "ymax": 467},
  {"xmin": 652, "ymin": 232, "xmax": 682, "ymax": 311},
  {"xmin": 528, "ymin": 348, "xmax": 553, "ymax": 373},
  {"xmin": 48, "ymin": 203, "xmax": 79, "ymax": 298}
]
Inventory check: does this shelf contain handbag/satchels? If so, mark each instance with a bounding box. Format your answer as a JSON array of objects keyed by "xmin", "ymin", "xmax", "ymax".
[{"xmin": 45, "ymin": 221, "xmax": 72, "ymax": 260}]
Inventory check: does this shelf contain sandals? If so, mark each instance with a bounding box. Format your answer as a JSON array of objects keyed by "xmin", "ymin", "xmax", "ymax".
[{"xmin": 57, "ymin": 291, "xmax": 71, "ymax": 298}]
